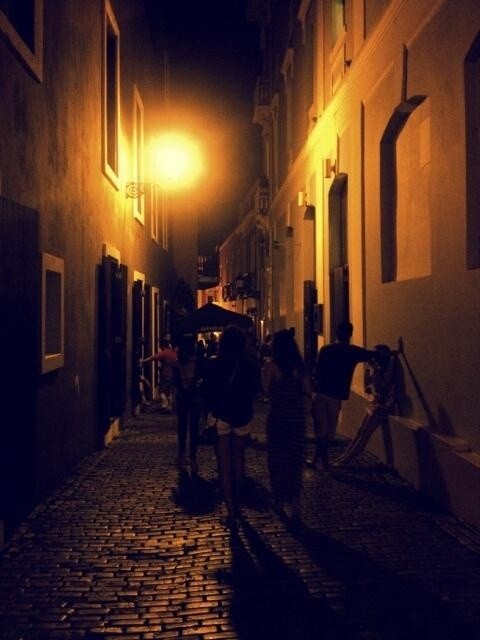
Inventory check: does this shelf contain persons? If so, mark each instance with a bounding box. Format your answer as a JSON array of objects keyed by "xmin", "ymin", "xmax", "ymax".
[{"xmin": 138, "ymin": 321, "xmax": 402, "ymax": 525}]
